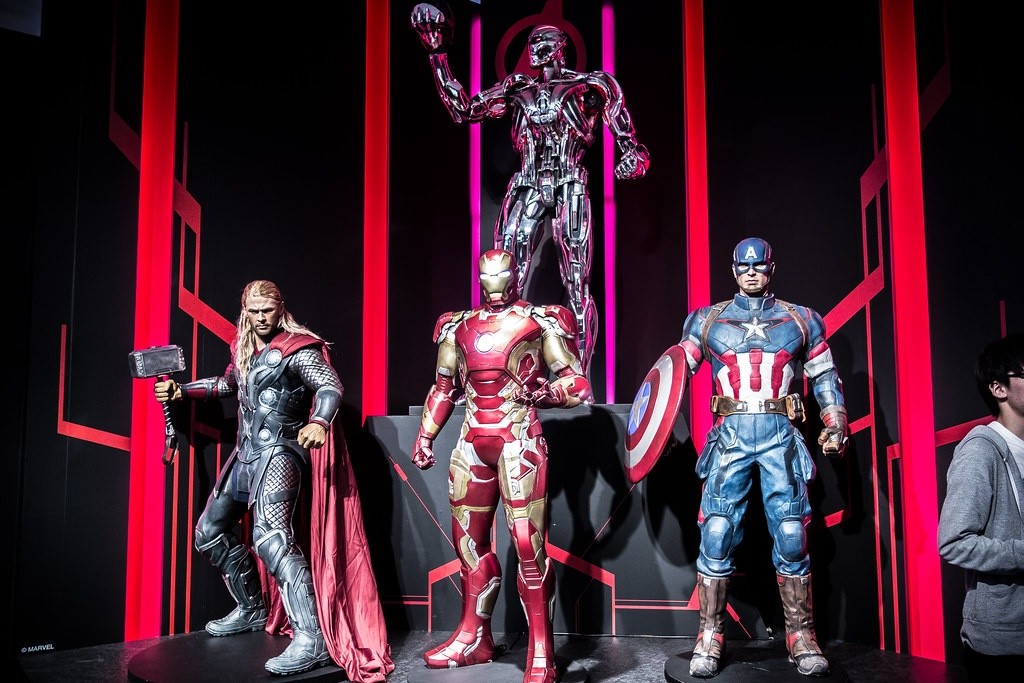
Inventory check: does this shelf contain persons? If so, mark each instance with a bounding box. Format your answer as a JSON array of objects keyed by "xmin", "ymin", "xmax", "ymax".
[
  {"xmin": 412, "ymin": 251, "xmax": 592, "ymax": 683},
  {"xmin": 411, "ymin": 4, "xmax": 651, "ymax": 406},
  {"xmin": 623, "ymin": 238, "xmax": 848, "ymax": 677},
  {"xmin": 128, "ymin": 279, "xmax": 343, "ymax": 675},
  {"xmin": 936, "ymin": 333, "xmax": 1024, "ymax": 681}
]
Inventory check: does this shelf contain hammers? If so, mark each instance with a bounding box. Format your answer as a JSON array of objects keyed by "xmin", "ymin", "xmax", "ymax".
[{"xmin": 128, "ymin": 344, "xmax": 185, "ymax": 449}]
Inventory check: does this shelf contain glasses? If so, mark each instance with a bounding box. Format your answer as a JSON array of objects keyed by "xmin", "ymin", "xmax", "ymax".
[{"xmin": 1009, "ymin": 373, "xmax": 1024, "ymax": 379}]
